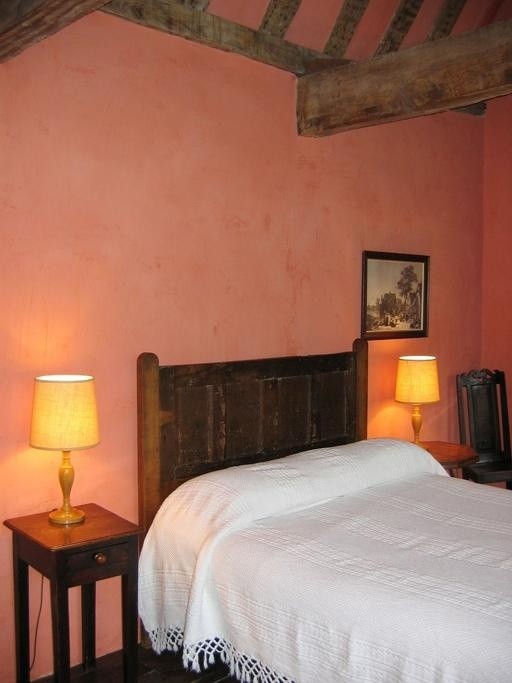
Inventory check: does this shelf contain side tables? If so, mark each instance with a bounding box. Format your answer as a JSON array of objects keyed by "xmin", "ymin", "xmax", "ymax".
[{"xmin": 408, "ymin": 441, "xmax": 479, "ymax": 478}]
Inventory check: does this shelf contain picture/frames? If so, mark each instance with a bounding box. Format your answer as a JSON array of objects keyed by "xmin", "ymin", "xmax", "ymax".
[{"xmin": 361, "ymin": 250, "xmax": 429, "ymax": 340}]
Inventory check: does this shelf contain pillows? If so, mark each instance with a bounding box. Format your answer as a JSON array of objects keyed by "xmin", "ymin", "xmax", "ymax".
[{"xmin": 157, "ymin": 437, "xmax": 448, "ymax": 537}]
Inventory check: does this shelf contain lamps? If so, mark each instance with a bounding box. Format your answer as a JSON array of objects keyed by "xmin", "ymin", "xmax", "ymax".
[
  {"xmin": 28, "ymin": 372, "xmax": 100, "ymax": 527},
  {"xmin": 394, "ymin": 355, "xmax": 441, "ymax": 450}
]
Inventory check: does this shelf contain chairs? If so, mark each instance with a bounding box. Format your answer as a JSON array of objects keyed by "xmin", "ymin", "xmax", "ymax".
[{"xmin": 455, "ymin": 369, "xmax": 512, "ymax": 490}]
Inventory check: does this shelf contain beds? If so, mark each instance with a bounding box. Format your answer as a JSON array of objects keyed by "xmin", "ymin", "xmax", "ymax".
[{"xmin": 134, "ymin": 337, "xmax": 512, "ymax": 682}]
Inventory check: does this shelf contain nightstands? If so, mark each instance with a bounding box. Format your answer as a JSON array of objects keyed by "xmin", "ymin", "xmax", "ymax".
[{"xmin": 2, "ymin": 503, "xmax": 146, "ymax": 683}]
